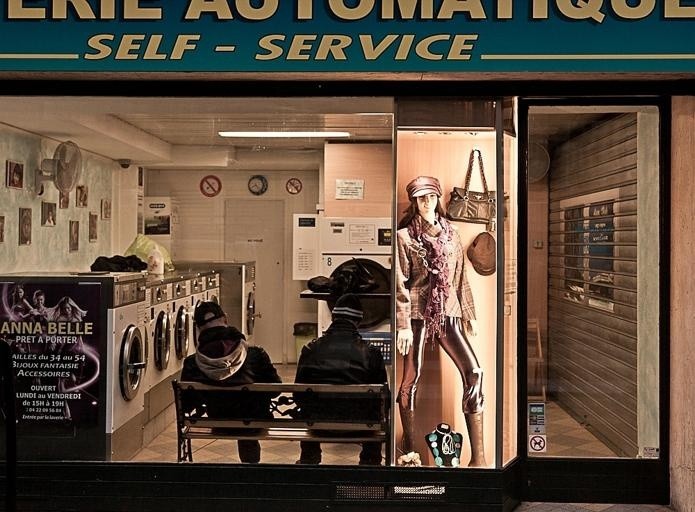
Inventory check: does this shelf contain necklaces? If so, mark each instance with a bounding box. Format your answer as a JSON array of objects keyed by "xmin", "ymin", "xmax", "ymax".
[
  {"xmin": 426, "ymin": 423, "xmax": 464, "ymax": 468},
  {"xmin": 417, "ymin": 218, "xmax": 452, "ymax": 276}
]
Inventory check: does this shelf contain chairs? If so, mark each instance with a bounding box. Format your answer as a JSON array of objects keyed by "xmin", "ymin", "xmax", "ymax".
[{"xmin": 527, "ymin": 311, "xmax": 548, "ymax": 402}]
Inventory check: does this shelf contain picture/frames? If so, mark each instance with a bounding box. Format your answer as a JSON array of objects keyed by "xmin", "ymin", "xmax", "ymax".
[{"xmin": 0, "ymin": 160, "xmax": 114, "ymax": 253}]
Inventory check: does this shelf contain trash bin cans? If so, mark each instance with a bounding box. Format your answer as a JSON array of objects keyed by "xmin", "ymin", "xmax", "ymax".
[{"xmin": 294, "ymin": 322, "xmax": 317, "ymax": 366}]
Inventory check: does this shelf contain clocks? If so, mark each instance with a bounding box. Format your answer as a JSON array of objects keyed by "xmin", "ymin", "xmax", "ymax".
[{"xmin": 246, "ymin": 172, "xmax": 270, "ymax": 197}]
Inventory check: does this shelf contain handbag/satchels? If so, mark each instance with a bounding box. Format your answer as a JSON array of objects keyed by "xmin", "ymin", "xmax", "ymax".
[{"xmin": 447, "ymin": 187, "xmax": 496, "ymax": 224}]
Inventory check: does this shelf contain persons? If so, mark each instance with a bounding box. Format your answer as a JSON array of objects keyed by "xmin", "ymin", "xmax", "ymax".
[
  {"xmin": 180, "ymin": 298, "xmax": 282, "ymax": 463},
  {"xmin": 9, "ymin": 286, "xmax": 88, "ymax": 425},
  {"xmin": 0, "ymin": 159, "xmax": 111, "ymax": 253},
  {"xmin": 398, "ymin": 175, "xmax": 488, "ymax": 468},
  {"xmin": 295, "ymin": 293, "xmax": 384, "ymax": 467}
]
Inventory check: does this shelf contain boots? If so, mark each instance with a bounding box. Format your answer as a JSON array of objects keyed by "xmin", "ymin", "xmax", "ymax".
[
  {"xmin": 400, "ymin": 411, "xmax": 416, "ymax": 452},
  {"xmin": 464, "ymin": 412, "xmax": 486, "ymax": 468}
]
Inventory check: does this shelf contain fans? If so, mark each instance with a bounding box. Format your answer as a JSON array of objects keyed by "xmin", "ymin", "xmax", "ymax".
[{"xmin": 28, "ymin": 134, "xmax": 85, "ymax": 204}]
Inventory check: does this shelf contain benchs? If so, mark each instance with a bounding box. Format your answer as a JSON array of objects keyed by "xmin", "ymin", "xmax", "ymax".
[{"xmin": 170, "ymin": 378, "xmax": 391, "ymax": 464}]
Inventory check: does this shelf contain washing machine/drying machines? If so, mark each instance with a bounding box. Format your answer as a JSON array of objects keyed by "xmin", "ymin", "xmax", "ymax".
[
  {"xmin": 0, "ymin": 270, "xmax": 149, "ymax": 463},
  {"xmin": 144, "ymin": 279, "xmax": 152, "ymax": 449},
  {"xmin": 192, "ymin": 276, "xmax": 208, "ymax": 354},
  {"xmin": 173, "ymin": 280, "xmax": 193, "ymax": 373},
  {"xmin": 151, "ymin": 282, "xmax": 173, "ymax": 389},
  {"xmin": 207, "ymin": 273, "xmax": 221, "ymax": 305},
  {"xmin": 172, "ymin": 258, "xmax": 263, "ymax": 342}
]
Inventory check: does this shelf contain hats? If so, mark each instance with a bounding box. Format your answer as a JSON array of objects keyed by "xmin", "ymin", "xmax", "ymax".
[
  {"xmin": 469, "ymin": 233, "xmax": 496, "ymax": 275},
  {"xmin": 195, "ymin": 302, "xmax": 224, "ymax": 326},
  {"xmin": 407, "ymin": 177, "xmax": 442, "ymax": 200},
  {"xmin": 331, "ymin": 293, "xmax": 364, "ymax": 321}
]
